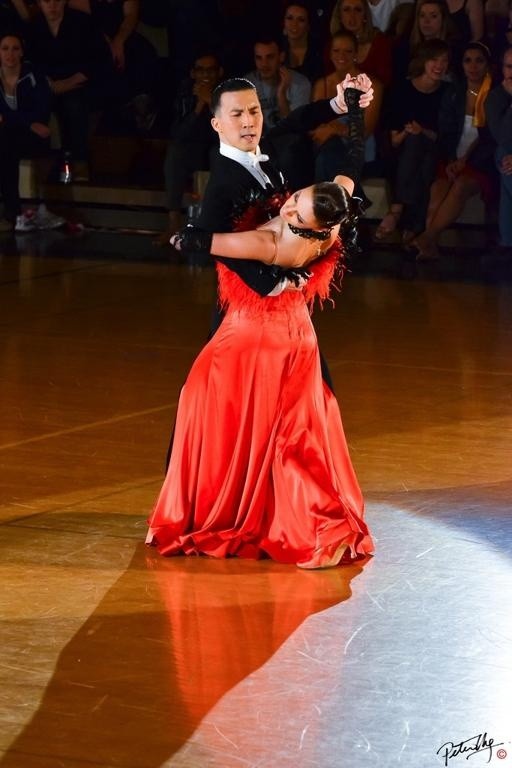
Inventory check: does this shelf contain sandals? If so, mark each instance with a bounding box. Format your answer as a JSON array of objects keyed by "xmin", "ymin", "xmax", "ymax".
[{"xmin": 375, "ymin": 207, "xmax": 441, "ymax": 260}]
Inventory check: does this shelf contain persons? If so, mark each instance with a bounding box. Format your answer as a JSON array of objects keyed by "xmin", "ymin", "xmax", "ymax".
[
  {"xmin": 145, "ymin": 72, "xmax": 374, "ymax": 570},
  {"xmin": 0, "ymin": 0, "xmax": 512, "ymax": 260},
  {"xmin": 165, "ymin": 73, "xmax": 374, "ymax": 477}
]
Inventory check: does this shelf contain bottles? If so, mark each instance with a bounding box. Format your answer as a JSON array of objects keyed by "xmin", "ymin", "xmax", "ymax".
[
  {"xmin": 59, "ymin": 150, "xmax": 73, "ymax": 185},
  {"xmin": 185, "ymin": 193, "xmax": 203, "ymax": 225}
]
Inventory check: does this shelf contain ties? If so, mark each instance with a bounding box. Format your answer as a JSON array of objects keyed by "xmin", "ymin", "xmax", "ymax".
[{"xmin": 249, "ymin": 153, "xmax": 269, "ymax": 169}]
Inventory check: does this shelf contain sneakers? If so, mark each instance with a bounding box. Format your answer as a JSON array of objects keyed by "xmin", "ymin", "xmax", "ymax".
[{"xmin": 15, "ymin": 207, "xmax": 66, "ymax": 232}]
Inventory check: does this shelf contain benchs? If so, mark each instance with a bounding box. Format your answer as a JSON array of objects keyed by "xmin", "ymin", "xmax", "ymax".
[{"xmin": 18, "ymin": 136, "xmax": 498, "ymax": 248}]
